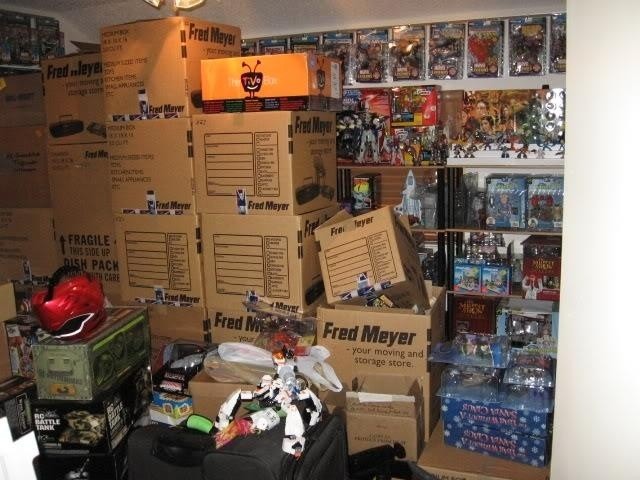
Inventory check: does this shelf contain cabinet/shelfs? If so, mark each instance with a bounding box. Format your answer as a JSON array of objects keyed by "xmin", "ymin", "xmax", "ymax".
[{"xmin": 446, "ymin": 156, "xmax": 560, "ymax": 303}]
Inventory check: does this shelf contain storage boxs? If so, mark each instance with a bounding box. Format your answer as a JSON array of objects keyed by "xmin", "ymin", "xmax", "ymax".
[
  {"xmin": 0, "ymin": 72, "xmax": 45, "ymax": 127},
  {"xmin": 1, "ymin": 282, "xmax": 150, "ymax": 479},
  {"xmin": 201, "ymin": 206, "xmax": 340, "ymax": 308},
  {"xmin": 199, "ymin": 50, "xmax": 344, "ymax": 115},
  {"xmin": 45, "ymin": 142, "xmax": 121, "ymax": 297},
  {"xmin": 313, "ymin": 284, "xmax": 445, "ymax": 444},
  {"xmin": 1, "ymin": 206, "xmax": 56, "ymax": 282},
  {"xmin": 342, "ymin": 377, "xmax": 427, "ymax": 463},
  {"xmin": 1, "ymin": 126, "xmax": 52, "ymax": 207},
  {"xmin": 193, "ymin": 111, "xmax": 336, "ymax": 215},
  {"xmin": 122, "ymin": 297, "xmax": 210, "ymax": 346},
  {"xmin": 41, "ymin": 40, "xmax": 113, "ymax": 145},
  {"xmin": 105, "ymin": 115, "xmax": 199, "ymax": 217},
  {"xmin": 208, "ymin": 308, "xmax": 317, "ymax": 348},
  {"xmin": 312, "ymin": 202, "xmax": 431, "ymax": 308},
  {"xmin": 112, "ymin": 214, "xmax": 206, "ymax": 309},
  {"xmin": 97, "ymin": 19, "xmax": 241, "ymax": 121}
]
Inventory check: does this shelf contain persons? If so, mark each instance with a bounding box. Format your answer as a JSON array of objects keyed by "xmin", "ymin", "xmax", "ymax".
[{"xmin": 336, "ymin": 110, "xmax": 565, "ymax": 167}]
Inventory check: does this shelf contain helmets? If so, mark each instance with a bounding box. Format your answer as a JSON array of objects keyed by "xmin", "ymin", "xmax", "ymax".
[{"xmin": 31, "ymin": 275, "xmax": 106, "ymax": 342}]
